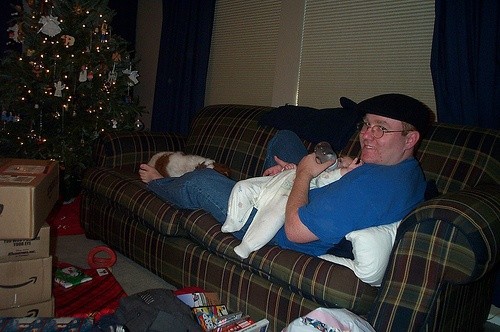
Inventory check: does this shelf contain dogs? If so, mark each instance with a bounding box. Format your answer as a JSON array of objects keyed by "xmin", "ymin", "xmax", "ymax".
[{"xmin": 132, "ymin": 150, "xmax": 228, "ymax": 184}]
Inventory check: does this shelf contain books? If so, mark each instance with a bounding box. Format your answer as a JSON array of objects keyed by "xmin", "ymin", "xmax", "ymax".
[
  {"xmin": 177, "ymin": 293, "xmax": 269, "ymax": 332},
  {"xmin": 55, "ymin": 267, "xmax": 92, "ymax": 288}
]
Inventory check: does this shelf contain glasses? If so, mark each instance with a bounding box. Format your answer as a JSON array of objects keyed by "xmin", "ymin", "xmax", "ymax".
[{"xmin": 357, "ymin": 122, "xmax": 414, "ymax": 139}]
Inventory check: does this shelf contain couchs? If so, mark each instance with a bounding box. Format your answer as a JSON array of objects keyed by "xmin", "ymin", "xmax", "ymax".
[{"xmin": 80, "ymin": 104, "xmax": 499, "ymax": 332}]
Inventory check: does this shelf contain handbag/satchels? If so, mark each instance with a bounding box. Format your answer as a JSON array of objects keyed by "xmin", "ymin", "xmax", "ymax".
[{"xmin": 281, "ymin": 307, "xmax": 376, "ymax": 332}]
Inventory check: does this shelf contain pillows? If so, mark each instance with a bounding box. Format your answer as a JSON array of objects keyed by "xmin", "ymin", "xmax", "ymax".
[{"xmin": 317, "ymin": 220, "xmax": 401, "ymax": 288}]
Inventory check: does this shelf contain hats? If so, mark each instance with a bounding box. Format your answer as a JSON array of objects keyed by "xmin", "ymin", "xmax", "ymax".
[{"xmin": 340, "ymin": 94, "xmax": 436, "ymax": 128}]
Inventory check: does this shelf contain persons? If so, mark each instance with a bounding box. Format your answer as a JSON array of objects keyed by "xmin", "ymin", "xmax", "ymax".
[
  {"xmin": 138, "ymin": 94, "xmax": 436, "ymax": 254},
  {"xmin": 221, "ymin": 152, "xmax": 363, "ymax": 259}
]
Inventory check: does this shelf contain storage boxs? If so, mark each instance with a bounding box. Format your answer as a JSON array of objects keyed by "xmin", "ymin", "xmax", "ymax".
[{"xmin": 0, "ymin": 157, "xmax": 59, "ymax": 319}]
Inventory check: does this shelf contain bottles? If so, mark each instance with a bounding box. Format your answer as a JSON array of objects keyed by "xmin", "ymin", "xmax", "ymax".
[{"xmin": 314, "ymin": 141, "xmax": 339, "ymax": 171}]
